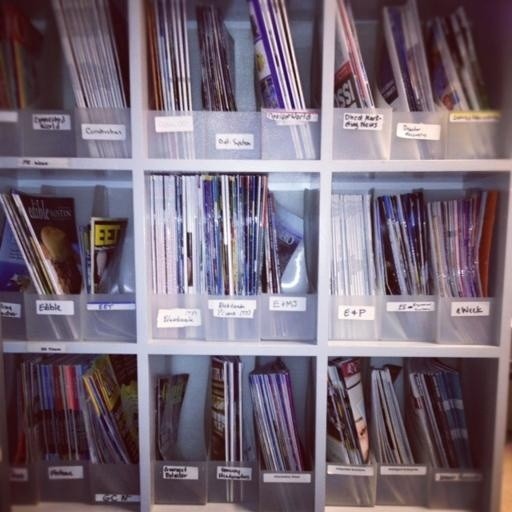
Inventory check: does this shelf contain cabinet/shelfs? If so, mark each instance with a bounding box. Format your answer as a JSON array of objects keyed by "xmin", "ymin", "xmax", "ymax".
[{"xmin": 0, "ymin": 1, "xmax": 511, "ymax": 512}]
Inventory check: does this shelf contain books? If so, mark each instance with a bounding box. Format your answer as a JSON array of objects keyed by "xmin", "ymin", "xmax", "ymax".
[
  {"xmin": 331, "ymin": 186, "xmax": 496, "ymax": 343},
  {"xmin": 327, "ymin": 357, "xmax": 476, "ymax": 506},
  {"xmin": 148, "ymin": 173, "xmax": 313, "ymax": 341},
  {"xmin": 156, "ymin": 356, "xmax": 311, "ymax": 511},
  {"xmin": 146, "ymin": 0, "xmax": 316, "ymax": 161},
  {"xmin": 1, "ymin": 1, "xmax": 130, "ymax": 160},
  {"xmin": 11, "ymin": 355, "xmax": 135, "ymax": 466},
  {"xmin": 0, "ymin": 187, "xmax": 135, "ymax": 341},
  {"xmin": 336, "ymin": 1, "xmax": 500, "ymax": 159}
]
